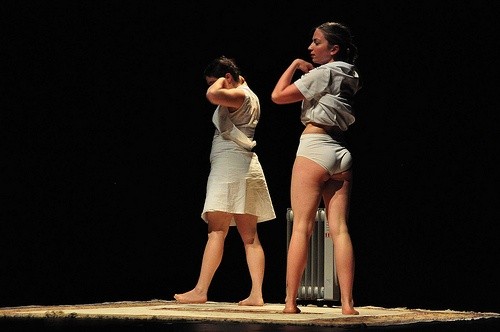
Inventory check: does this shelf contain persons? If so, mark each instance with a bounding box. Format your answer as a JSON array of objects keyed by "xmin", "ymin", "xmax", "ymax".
[
  {"xmin": 173, "ymin": 56, "xmax": 276, "ymax": 305},
  {"xmin": 272, "ymin": 22, "xmax": 361, "ymax": 315}
]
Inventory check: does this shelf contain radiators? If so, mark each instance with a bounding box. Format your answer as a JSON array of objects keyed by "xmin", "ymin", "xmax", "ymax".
[{"xmin": 286, "ymin": 206, "xmax": 355, "ymax": 309}]
